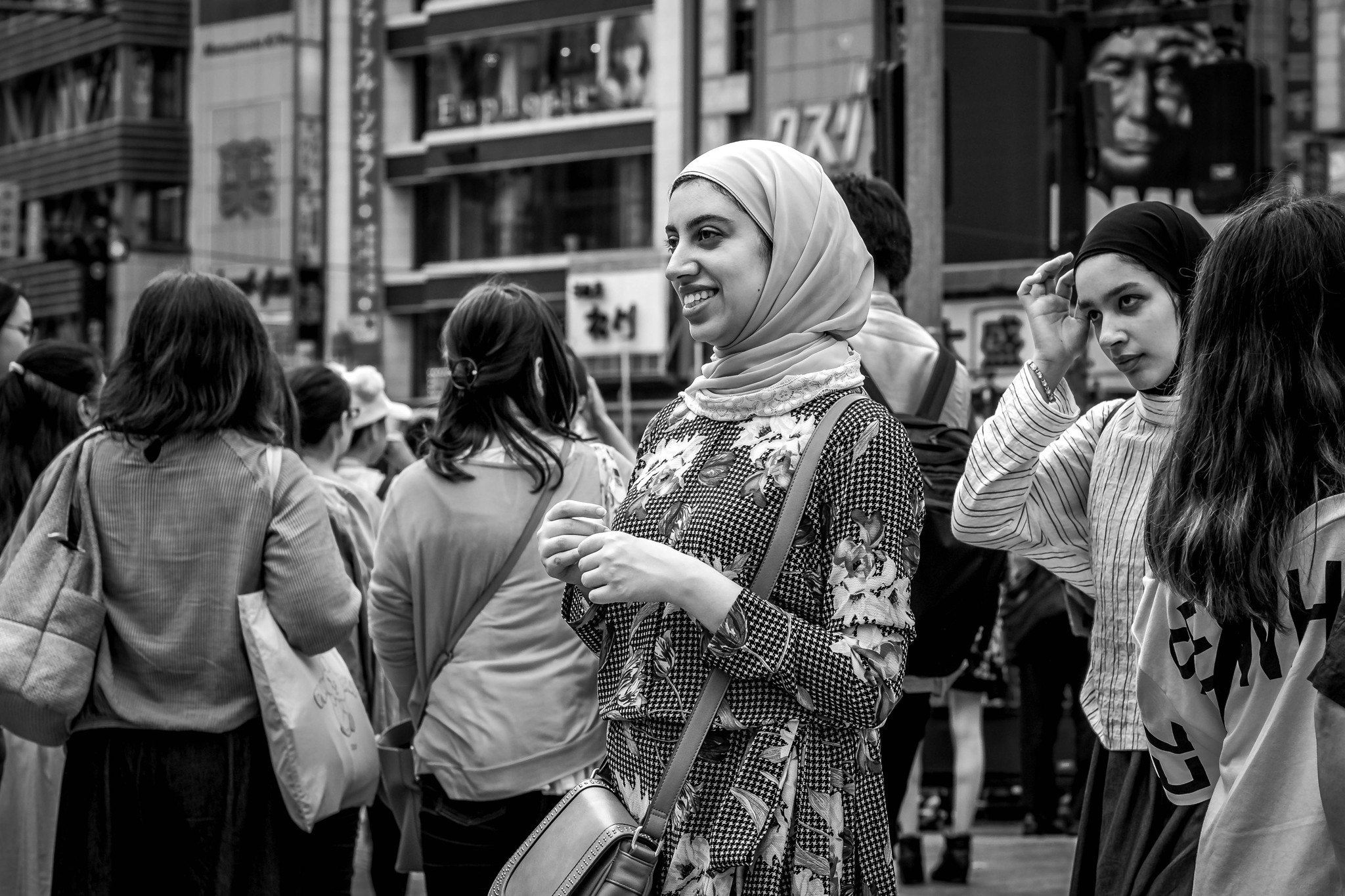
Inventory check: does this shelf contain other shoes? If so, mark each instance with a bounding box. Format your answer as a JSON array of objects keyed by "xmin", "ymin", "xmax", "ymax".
[
  {"xmin": 1025, "ymin": 815, "xmax": 1062, "ymax": 835},
  {"xmin": 1063, "ymin": 817, "xmax": 1080, "ymax": 835}
]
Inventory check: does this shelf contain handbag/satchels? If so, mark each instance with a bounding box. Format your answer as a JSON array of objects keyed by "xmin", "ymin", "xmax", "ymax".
[
  {"xmin": 237, "ymin": 446, "xmax": 380, "ymax": 833},
  {"xmin": 0, "ymin": 425, "xmax": 106, "ymax": 746},
  {"xmin": 377, "ymin": 719, "xmax": 423, "ymax": 872},
  {"xmin": 486, "ymin": 778, "xmax": 659, "ymax": 896}
]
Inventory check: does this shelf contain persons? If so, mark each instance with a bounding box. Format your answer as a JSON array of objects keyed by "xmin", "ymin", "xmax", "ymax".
[
  {"xmin": 829, "ymin": 173, "xmax": 1095, "ymax": 884},
  {"xmin": 537, "ymin": 139, "xmax": 926, "ymax": 896},
  {"xmin": 1131, "ymin": 191, "xmax": 1345, "ymax": 896},
  {"xmin": 0, "ymin": 269, "xmax": 638, "ymax": 896},
  {"xmin": 1055, "ymin": 0, "xmax": 1223, "ymax": 252},
  {"xmin": 950, "ymin": 201, "xmax": 1215, "ymax": 896}
]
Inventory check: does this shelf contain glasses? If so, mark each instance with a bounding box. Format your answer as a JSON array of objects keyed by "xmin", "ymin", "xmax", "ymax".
[
  {"xmin": 5, "ymin": 323, "xmax": 38, "ymax": 346},
  {"xmin": 347, "ymin": 407, "xmax": 360, "ymax": 418}
]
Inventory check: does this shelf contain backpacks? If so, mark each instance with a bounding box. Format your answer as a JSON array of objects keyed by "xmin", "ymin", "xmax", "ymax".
[{"xmin": 852, "ymin": 347, "xmax": 1006, "ymax": 678}]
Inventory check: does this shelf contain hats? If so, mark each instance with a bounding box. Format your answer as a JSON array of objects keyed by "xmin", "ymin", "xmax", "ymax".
[{"xmin": 354, "ymin": 389, "xmax": 413, "ymax": 430}]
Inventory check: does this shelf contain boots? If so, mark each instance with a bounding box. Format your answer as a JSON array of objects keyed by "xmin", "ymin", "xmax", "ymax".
[
  {"xmin": 897, "ymin": 834, "xmax": 924, "ymax": 884},
  {"xmin": 929, "ymin": 834, "xmax": 973, "ymax": 883}
]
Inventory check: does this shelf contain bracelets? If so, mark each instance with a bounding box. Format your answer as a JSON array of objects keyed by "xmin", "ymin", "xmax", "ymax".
[{"xmin": 1026, "ymin": 360, "xmax": 1056, "ymax": 403}]
